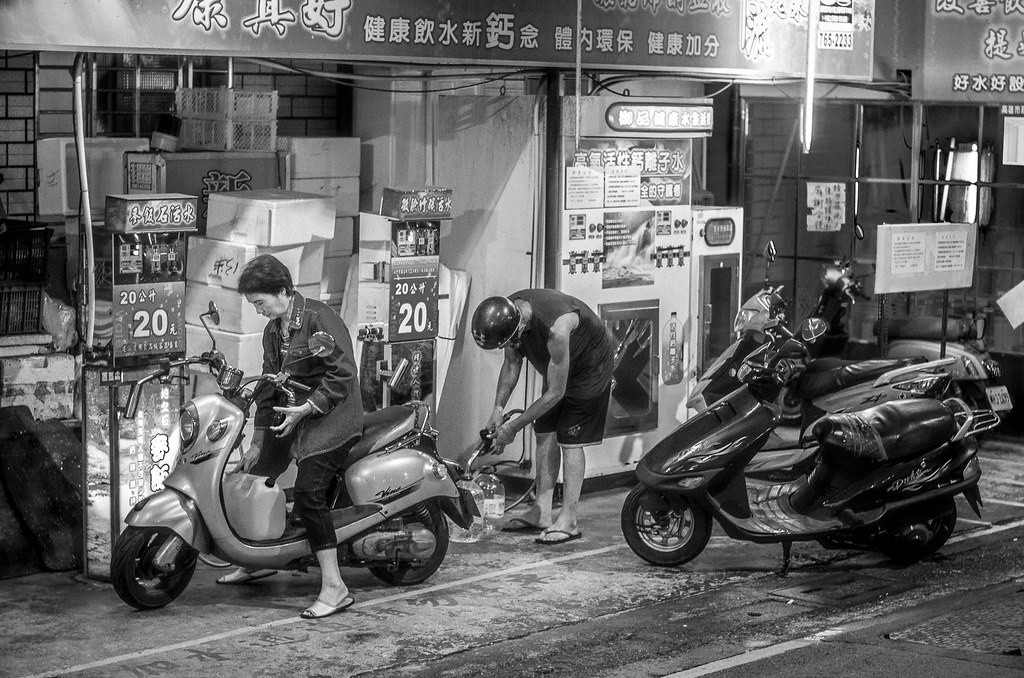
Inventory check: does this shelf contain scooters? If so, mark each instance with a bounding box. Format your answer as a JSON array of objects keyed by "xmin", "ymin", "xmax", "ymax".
[
  {"xmin": 109, "ymin": 301, "xmax": 482, "ymax": 612},
  {"xmin": 620, "ymin": 240, "xmax": 1001, "ymax": 577}
]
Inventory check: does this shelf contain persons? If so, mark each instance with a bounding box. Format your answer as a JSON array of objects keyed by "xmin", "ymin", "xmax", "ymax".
[
  {"xmin": 472, "ymin": 288, "xmax": 614, "ymax": 543},
  {"xmin": 216, "ymin": 255, "xmax": 364, "ymax": 619}
]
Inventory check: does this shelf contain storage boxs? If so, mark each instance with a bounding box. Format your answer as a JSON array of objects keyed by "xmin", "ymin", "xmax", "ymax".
[{"xmin": 0, "ymin": 84, "xmax": 451, "ymax": 580}]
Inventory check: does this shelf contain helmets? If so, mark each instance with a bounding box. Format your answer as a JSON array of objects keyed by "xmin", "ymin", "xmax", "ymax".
[{"xmin": 471, "ymin": 297, "xmax": 522, "ymax": 351}]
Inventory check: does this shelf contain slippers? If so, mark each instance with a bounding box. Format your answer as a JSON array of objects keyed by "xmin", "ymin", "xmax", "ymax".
[
  {"xmin": 501, "ymin": 519, "xmax": 545, "ymax": 532},
  {"xmin": 301, "ymin": 597, "xmax": 354, "ymax": 618},
  {"xmin": 535, "ymin": 530, "xmax": 582, "ymax": 544},
  {"xmin": 216, "ymin": 569, "xmax": 277, "ymax": 585}
]
